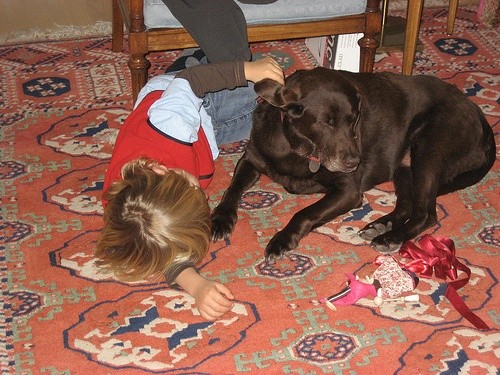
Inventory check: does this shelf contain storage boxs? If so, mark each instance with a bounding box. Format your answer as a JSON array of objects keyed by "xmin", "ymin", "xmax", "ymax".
[{"xmin": 305, "ymin": 33, "xmax": 365, "ymax": 73}]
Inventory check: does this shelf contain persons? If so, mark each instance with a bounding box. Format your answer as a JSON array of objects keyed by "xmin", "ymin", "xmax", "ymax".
[{"xmin": 94, "ymin": 50, "xmax": 285, "ymax": 320}]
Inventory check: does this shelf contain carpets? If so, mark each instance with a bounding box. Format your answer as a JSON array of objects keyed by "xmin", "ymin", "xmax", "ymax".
[{"xmin": 0, "ymin": 5, "xmax": 500, "ymax": 374}]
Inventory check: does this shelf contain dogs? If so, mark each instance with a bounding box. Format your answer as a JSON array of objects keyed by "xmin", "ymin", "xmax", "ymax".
[{"xmin": 209, "ymin": 66, "xmax": 498, "ymax": 267}]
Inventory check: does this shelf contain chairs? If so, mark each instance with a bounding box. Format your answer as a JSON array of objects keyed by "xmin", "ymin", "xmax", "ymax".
[{"xmin": 111, "ymin": 0, "xmax": 383, "ymax": 109}]
